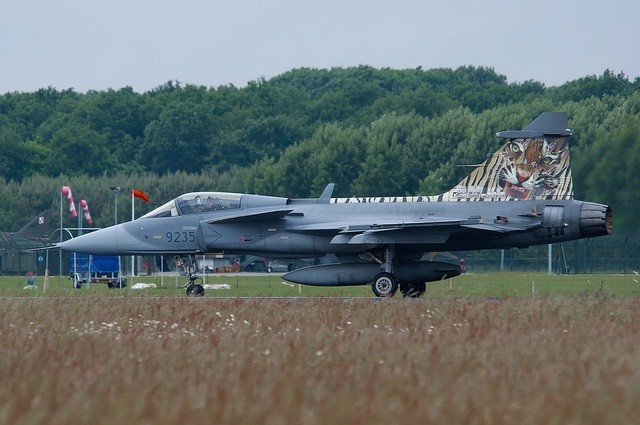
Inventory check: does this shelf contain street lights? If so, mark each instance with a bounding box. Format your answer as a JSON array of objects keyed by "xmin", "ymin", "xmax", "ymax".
[{"xmin": 110, "ymin": 186, "xmax": 120, "ymax": 225}]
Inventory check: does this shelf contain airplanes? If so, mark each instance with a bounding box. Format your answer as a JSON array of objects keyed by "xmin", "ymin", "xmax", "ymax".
[{"xmin": 57, "ymin": 111, "xmax": 612, "ymax": 297}]
